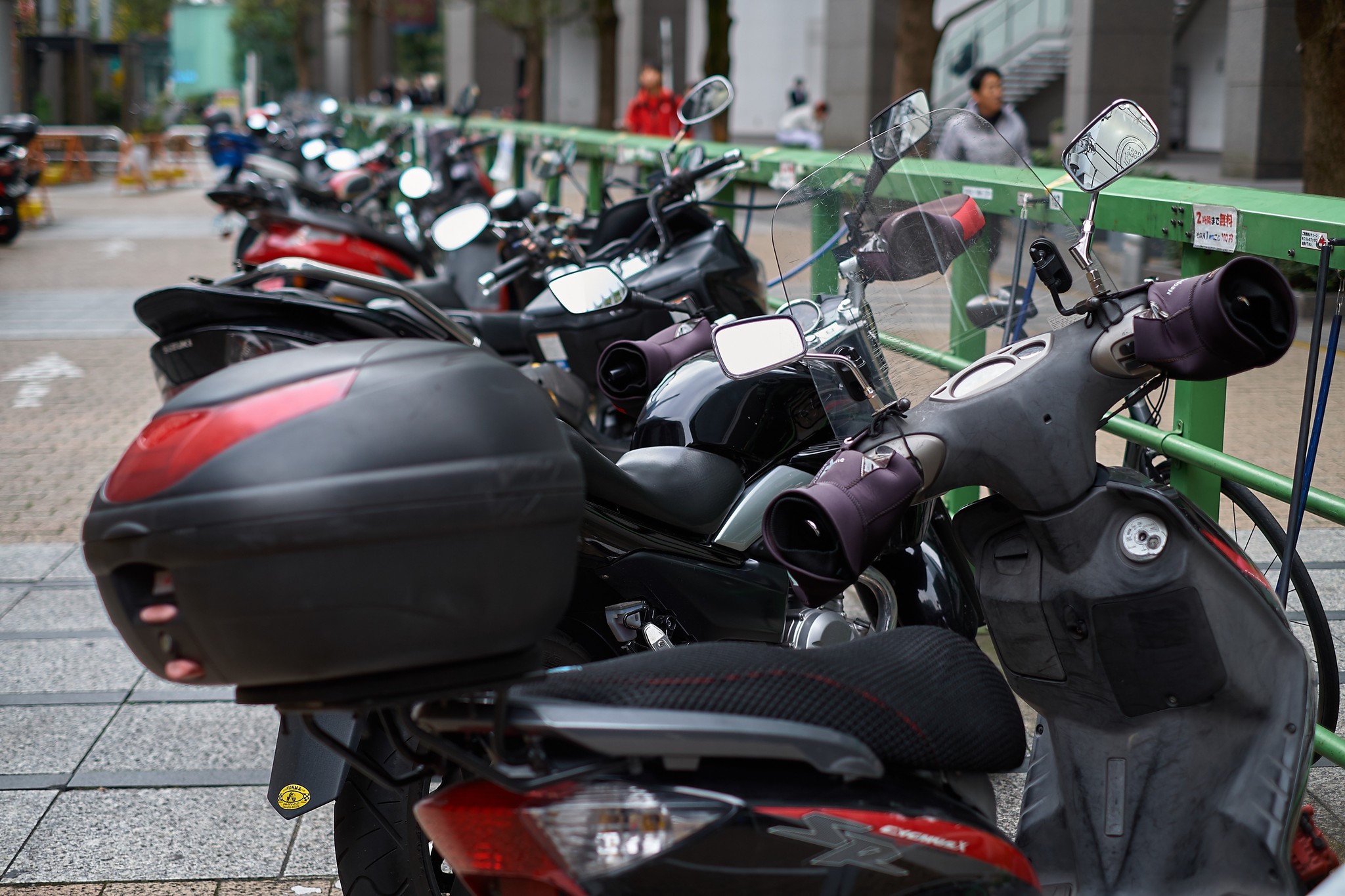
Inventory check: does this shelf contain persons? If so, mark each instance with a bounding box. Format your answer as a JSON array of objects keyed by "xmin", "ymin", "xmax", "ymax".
[
  {"xmin": 787, "ymin": 77, "xmax": 809, "ymax": 107},
  {"xmin": 775, "ymin": 98, "xmax": 830, "ymax": 150},
  {"xmin": 932, "ymin": 64, "xmax": 1031, "ymax": 266},
  {"xmin": 625, "ymin": 63, "xmax": 695, "ymax": 138}
]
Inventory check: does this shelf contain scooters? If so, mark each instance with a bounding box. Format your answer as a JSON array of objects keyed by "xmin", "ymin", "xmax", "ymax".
[
  {"xmin": 80, "ymin": 97, "xmax": 1345, "ymax": 896},
  {"xmin": 151, "ymin": 74, "xmax": 987, "ymax": 653},
  {"xmin": 1, "ymin": 110, "xmax": 47, "ymax": 249}
]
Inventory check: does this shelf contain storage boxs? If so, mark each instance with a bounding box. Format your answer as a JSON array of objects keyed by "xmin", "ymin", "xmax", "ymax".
[{"xmin": 83, "ymin": 343, "xmax": 586, "ymax": 683}]
